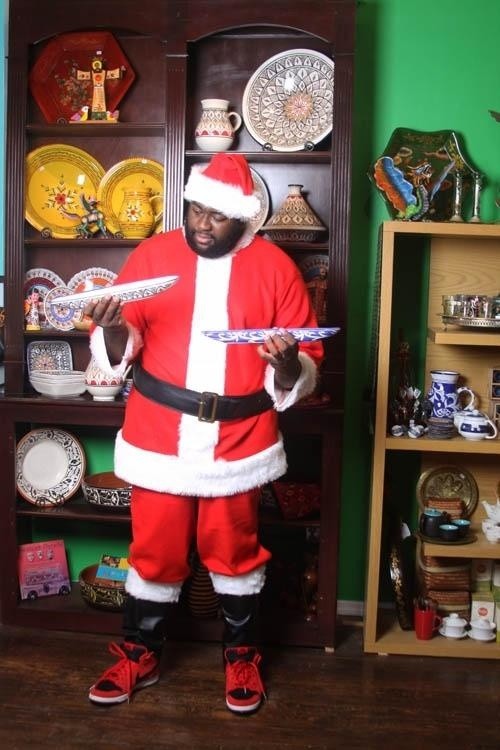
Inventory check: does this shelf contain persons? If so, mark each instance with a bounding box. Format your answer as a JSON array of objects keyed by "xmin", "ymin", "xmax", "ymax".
[{"xmin": 84, "ymin": 152, "xmax": 325, "ymax": 714}]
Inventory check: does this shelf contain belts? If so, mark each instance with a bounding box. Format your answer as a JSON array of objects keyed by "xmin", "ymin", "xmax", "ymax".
[{"xmin": 132, "ymin": 348, "xmax": 275, "ymax": 425}]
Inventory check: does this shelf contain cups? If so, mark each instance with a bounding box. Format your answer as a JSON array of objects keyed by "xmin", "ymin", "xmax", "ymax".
[
  {"xmin": 481, "ymin": 517, "xmax": 499, "ymax": 544},
  {"xmin": 418, "ymin": 508, "xmax": 471, "ymax": 541},
  {"xmin": 440, "ymin": 612, "xmax": 495, "ymax": 637},
  {"xmin": 449, "ymin": 171, "xmax": 466, "ymax": 222},
  {"xmin": 440, "ymin": 291, "xmax": 500, "ymax": 318},
  {"xmin": 412, "ymin": 607, "xmax": 441, "ymax": 640},
  {"xmin": 468, "ymin": 171, "xmax": 486, "ymax": 224}
]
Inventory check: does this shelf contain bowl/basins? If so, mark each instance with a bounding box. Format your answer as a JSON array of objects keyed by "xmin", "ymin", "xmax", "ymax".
[
  {"xmin": 122, "ymin": 378, "xmax": 133, "ymax": 395},
  {"xmin": 79, "ymin": 562, "xmax": 128, "ymax": 611},
  {"xmin": 87, "ymin": 375, "xmax": 125, "ymax": 403},
  {"xmin": 26, "ymin": 340, "xmax": 86, "ymax": 401},
  {"xmin": 80, "ymin": 471, "xmax": 131, "ymax": 514}
]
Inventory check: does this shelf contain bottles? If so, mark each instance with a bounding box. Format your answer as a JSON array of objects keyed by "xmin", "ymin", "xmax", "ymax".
[{"xmin": 261, "ymin": 183, "xmax": 328, "ymax": 243}]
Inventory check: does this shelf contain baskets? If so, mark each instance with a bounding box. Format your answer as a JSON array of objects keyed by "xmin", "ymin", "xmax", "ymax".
[
  {"xmin": 423, "ymin": 588, "xmax": 472, "ymax": 625},
  {"xmin": 420, "ymin": 549, "xmax": 473, "ymax": 591}
]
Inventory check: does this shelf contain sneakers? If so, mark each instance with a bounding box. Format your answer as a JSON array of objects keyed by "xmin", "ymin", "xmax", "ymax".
[
  {"xmin": 88, "ymin": 641, "xmax": 162, "ymax": 704},
  {"xmin": 222, "ymin": 646, "xmax": 264, "ymax": 713}
]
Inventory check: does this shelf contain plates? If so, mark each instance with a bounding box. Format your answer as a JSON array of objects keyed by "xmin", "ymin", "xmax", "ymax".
[
  {"xmin": 24, "ymin": 143, "xmax": 107, "ymax": 239},
  {"xmin": 25, "ymin": 31, "xmax": 140, "ymax": 123},
  {"xmin": 51, "ymin": 274, "xmax": 181, "ymax": 313},
  {"xmin": 198, "ymin": 327, "xmax": 342, "ymax": 347},
  {"xmin": 365, "ymin": 126, "xmax": 483, "ymax": 224},
  {"xmin": 242, "ymin": 47, "xmax": 335, "ymax": 155},
  {"xmin": 96, "ymin": 158, "xmax": 165, "ymax": 240},
  {"xmin": 16, "ymin": 427, "xmax": 87, "ymax": 507},
  {"xmin": 296, "ymin": 255, "xmax": 331, "ymax": 322},
  {"xmin": 24, "ymin": 268, "xmax": 118, "ymax": 332},
  {"xmin": 436, "ymin": 629, "xmax": 497, "ymax": 642}
]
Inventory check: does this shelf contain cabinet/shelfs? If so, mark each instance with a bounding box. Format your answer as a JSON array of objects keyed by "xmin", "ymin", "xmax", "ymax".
[
  {"xmin": 366, "ymin": 217, "xmax": 500, "ymax": 665},
  {"xmin": 7, "ymin": 1, "xmax": 354, "ymax": 653}
]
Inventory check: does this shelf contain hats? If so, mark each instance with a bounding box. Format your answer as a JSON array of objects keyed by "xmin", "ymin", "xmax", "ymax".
[{"xmin": 180, "ymin": 152, "xmax": 262, "ymax": 225}]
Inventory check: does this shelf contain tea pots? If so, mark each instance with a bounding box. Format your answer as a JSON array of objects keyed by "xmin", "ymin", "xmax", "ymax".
[
  {"xmin": 424, "ymin": 369, "xmax": 497, "ymax": 442},
  {"xmin": 481, "ymin": 498, "xmax": 500, "ymax": 523},
  {"xmin": 120, "ymin": 186, "xmax": 165, "ymax": 240},
  {"xmin": 195, "ymin": 97, "xmax": 242, "ymax": 155}
]
